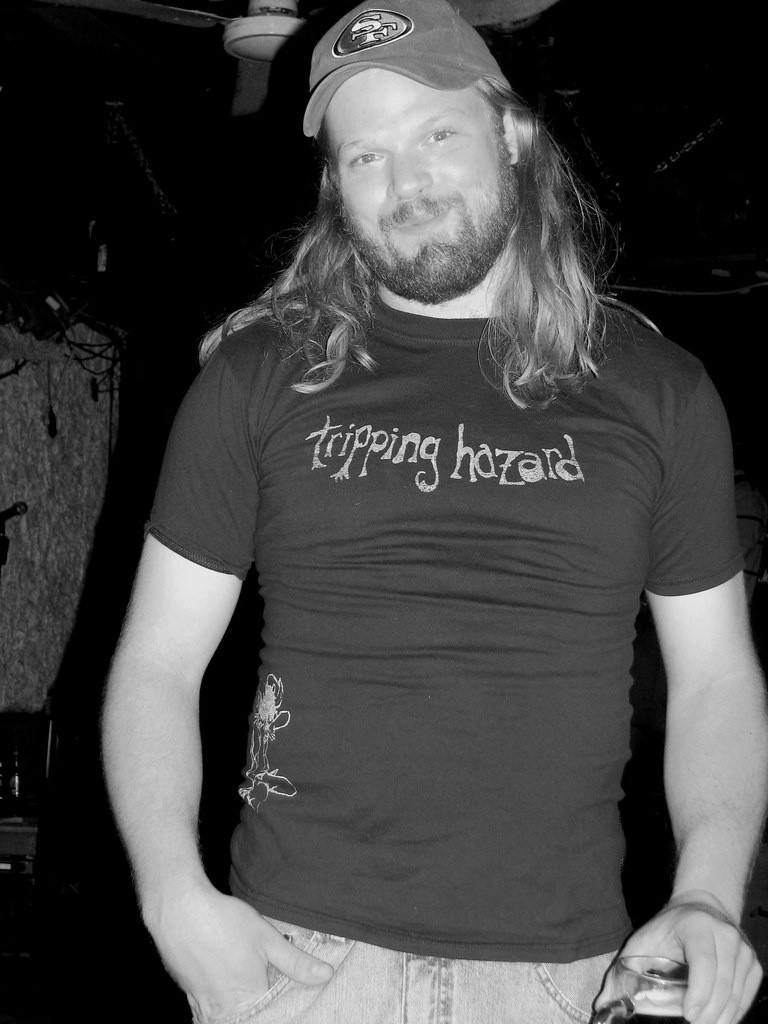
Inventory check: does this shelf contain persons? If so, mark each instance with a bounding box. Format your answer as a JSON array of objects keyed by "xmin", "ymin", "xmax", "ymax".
[{"xmin": 104, "ymin": 0, "xmax": 768, "ymax": 1024}]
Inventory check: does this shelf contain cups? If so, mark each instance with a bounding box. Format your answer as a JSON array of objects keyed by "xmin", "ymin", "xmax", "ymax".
[{"xmin": 593, "ymin": 956, "xmax": 690, "ymax": 1024}]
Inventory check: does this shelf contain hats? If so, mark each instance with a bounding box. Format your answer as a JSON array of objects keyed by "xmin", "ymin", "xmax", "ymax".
[{"xmin": 301, "ymin": 0, "xmax": 513, "ymax": 140}]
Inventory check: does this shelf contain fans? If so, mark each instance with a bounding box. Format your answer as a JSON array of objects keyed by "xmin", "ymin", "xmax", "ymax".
[{"xmin": 46, "ymin": 0, "xmax": 561, "ymax": 117}]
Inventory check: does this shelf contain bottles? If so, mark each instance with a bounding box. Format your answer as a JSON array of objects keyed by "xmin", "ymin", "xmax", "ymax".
[{"xmin": 10, "ymin": 742, "xmax": 27, "ymax": 799}]
